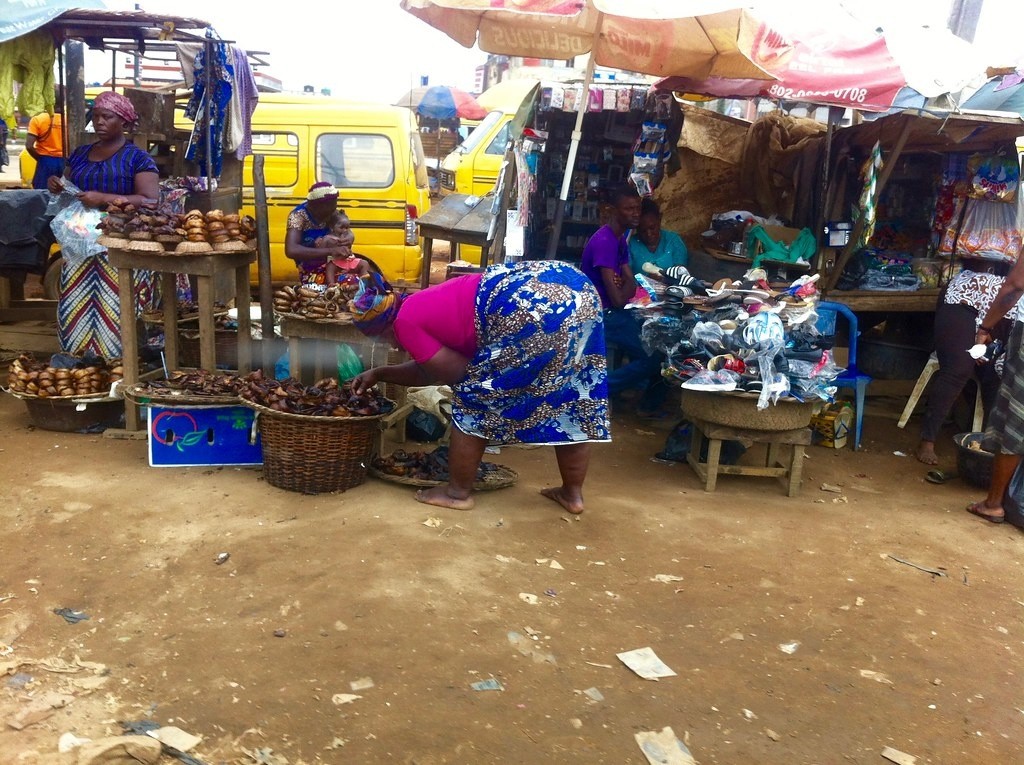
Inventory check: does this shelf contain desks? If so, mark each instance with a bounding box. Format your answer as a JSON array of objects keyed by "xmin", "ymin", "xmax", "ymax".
[
  {"xmin": 107, "ymin": 247, "xmax": 255, "ymax": 429},
  {"xmin": 413, "ymin": 193, "xmax": 498, "ymax": 291},
  {"xmin": 279, "ymin": 318, "xmax": 409, "ymax": 457},
  {"xmin": 821, "ymin": 287, "xmax": 944, "ymax": 402}
]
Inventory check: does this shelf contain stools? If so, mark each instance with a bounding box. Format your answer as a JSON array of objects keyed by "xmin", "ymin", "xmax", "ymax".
[{"xmin": 685, "ymin": 416, "xmax": 812, "ymax": 498}]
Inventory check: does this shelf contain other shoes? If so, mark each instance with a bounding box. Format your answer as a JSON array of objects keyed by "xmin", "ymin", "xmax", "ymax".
[{"xmin": 626, "ymin": 260, "xmax": 836, "ymax": 393}]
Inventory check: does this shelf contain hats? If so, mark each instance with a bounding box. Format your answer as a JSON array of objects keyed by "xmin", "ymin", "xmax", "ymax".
[
  {"xmin": 345, "ymin": 267, "xmax": 403, "ymax": 336},
  {"xmin": 307, "ymin": 182, "xmax": 339, "ymax": 205}
]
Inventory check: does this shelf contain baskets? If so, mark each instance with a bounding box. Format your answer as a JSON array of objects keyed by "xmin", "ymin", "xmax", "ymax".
[{"xmin": 261, "ymin": 412, "xmax": 381, "ymax": 494}]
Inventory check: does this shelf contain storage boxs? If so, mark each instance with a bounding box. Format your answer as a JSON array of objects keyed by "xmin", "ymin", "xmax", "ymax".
[{"xmin": 146, "ymin": 407, "xmax": 264, "ymax": 467}]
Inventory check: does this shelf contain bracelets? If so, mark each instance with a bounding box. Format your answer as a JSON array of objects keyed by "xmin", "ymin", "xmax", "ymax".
[{"xmin": 979, "ymin": 324, "xmax": 993, "ymax": 333}]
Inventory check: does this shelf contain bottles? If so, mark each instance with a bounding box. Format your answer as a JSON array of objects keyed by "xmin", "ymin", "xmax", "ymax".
[
  {"xmin": 735, "ymin": 215, "xmax": 744, "ymax": 222},
  {"xmin": 742, "ymin": 218, "xmax": 752, "ymax": 256},
  {"xmin": 641, "ymin": 262, "xmax": 664, "ymax": 276}
]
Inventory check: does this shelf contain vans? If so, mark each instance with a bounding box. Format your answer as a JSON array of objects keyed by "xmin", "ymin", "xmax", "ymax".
[
  {"xmin": 437, "ymin": 109, "xmax": 516, "ymax": 196},
  {"xmin": 18, "ymin": 89, "xmax": 430, "ymax": 289}
]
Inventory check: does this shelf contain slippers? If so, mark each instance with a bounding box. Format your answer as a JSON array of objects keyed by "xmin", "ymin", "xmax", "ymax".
[
  {"xmin": 924, "ymin": 468, "xmax": 959, "ymax": 484},
  {"xmin": 966, "ymin": 503, "xmax": 1006, "ymax": 522}
]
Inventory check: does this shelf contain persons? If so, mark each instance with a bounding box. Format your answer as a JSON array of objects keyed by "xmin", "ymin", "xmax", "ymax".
[
  {"xmin": 13, "ymin": 106, "xmax": 22, "ymax": 139},
  {"xmin": 0, "ymin": 115, "xmax": 9, "ymax": 173},
  {"xmin": 916, "ymin": 241, "xmax": 1024, "ymax": 523},
  {"xmin": 349, "ymin": 259, "xmax": 613, "ymax": 515},
  {"xmin": 579, "ymin": 185, "xmax": 688, "ymax": 397},
  {"xmin": 285, "ymin": 181, "xmax": 370, "ymax": 291},
  {"xmin": 26, "ymin": 84, "xmax": 68, "ymax": 188},
  {"xmin": 47, "ymin": 91, "xmax": 159, "ymax": 366}
]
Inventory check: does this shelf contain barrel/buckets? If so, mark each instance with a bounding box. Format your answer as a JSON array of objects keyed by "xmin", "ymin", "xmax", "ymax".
[{"xmin": 912, "ymin": 260, "xmax": 943, "ymax": 289}]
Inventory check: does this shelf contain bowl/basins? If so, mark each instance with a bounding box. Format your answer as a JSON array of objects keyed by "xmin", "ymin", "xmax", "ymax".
[
  {"xmin": 21, "ymin": 397, "xmax": 124, "ymax": 432},
  {"xmin": 731, "ymin": 242, "xmax": 742, "ymax": 254},
  {"xmin": 952, "ymin": 432, "xmax": 995, "ymax": 490}
]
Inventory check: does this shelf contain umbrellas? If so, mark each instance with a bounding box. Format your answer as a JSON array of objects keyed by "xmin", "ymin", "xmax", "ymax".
[
  {"xmin": 394, "ymin": 83, "xmax": 492, "ymax": 196},
  {"xmin": 399, "ymin": 0, "xmax": 795, "ymax": 261},
  {"xmin": 653, "ymin": 9, "xmax": 985, "ymax": 277}
]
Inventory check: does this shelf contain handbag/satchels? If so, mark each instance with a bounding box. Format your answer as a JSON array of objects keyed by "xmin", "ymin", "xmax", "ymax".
[{"xmin": 655, "ymin": 419, "xmax": 747, "ymax": 465}]
